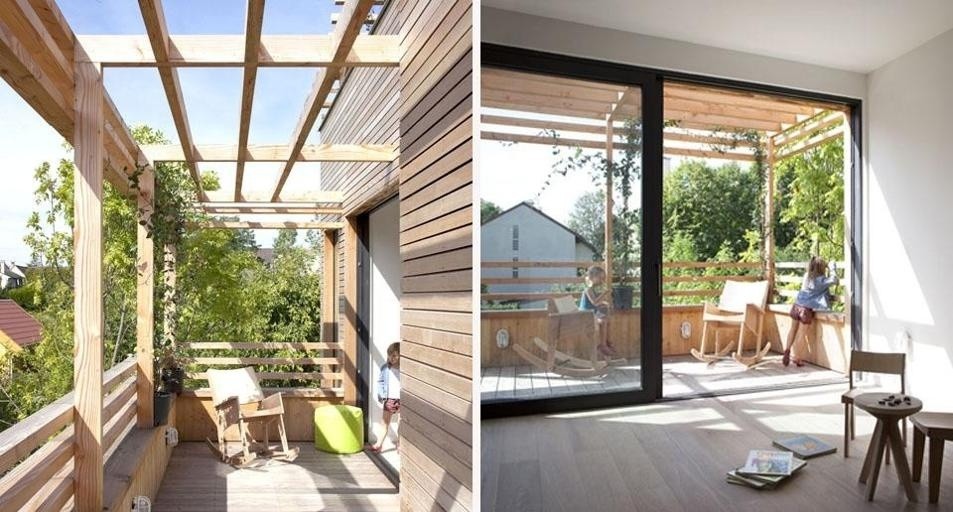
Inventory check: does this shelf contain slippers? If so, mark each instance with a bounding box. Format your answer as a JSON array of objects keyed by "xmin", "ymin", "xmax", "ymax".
[
  {"xmin": 783, "ymin": 350, "xmax": 790, "ymax": 366},
  {"xmin": 794, "ymin": 358, "xmax": 803, "ymax": 366}
]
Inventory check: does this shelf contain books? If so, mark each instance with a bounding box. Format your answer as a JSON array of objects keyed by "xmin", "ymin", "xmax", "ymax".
[{"xmin": 725, "ymin": 434, "xmax": 837, "ymax": 491}]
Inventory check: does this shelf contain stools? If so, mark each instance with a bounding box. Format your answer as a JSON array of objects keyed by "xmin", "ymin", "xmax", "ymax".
[{"xmin": 313, "ymin": 404, "xmax": 364, "ymax": 454}]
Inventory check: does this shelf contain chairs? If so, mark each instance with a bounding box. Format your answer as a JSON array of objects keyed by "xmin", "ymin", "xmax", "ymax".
[
  {"xmin": 840, "ymin": 350, "xmax": 952, "ymax": 503},
  {"xmin": 511, "ymin": 294, "xmax": 628, "ymax": 379},
  {"xmin": 204, "ymin": 365, "xmax": 299, "ymax": 469},
  {"xmin": 690, "ymin": 279, "xmax": 772, "ymax": 365}
]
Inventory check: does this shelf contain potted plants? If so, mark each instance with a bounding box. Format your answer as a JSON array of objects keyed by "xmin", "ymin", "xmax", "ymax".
[
  {"xmin": 590, "ymin": 204, "xmax": 643, "ymax": 311},
  {"xmin": 153, "ymin": 343, "xmax": 188, "ymax": 426}
]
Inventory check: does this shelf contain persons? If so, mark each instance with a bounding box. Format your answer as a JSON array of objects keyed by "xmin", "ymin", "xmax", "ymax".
[
  {"xmin": 578, "ymin": 266, "xmax": 617, "ymax": 356},
  {"xmin": 782, "ymin": 257, "xmax": 839, "ymax": 368},
  {"xmin": 363, "ymin": 343, "xmax": 400, "ymax": 456}
]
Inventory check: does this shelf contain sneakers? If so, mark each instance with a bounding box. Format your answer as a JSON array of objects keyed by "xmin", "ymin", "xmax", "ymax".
[
  {"xmin": 366, "ymin": 445, "xmax": 382, "ymax": 452},
  {"xmin": 597, "ymin": 341, "xmax": 616, "ymax": 355}
]
